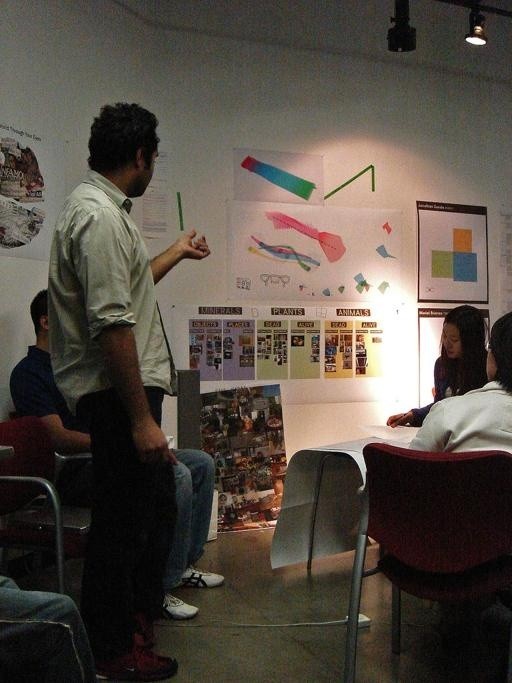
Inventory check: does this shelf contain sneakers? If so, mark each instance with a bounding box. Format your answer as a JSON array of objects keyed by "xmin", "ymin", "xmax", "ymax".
[
  {"xmin": 163, "ymin": 593, "xmax": 200, "ymax": 618},
  {"xmin": 132, "ymin": 620, "xmax": 155, "ymax": 650},
  {"xmin": 181, "ymin": 565, "xmax": 224, "ymax": 589},
  {"xmin": 96, "ymin": 647, "xmax": 179, "ymax": 681}
]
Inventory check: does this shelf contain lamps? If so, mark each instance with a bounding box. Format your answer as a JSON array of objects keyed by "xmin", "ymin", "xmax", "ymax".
[{"xmin": 385, "ymin": 0, "xmax": 492, "ymax": 56}]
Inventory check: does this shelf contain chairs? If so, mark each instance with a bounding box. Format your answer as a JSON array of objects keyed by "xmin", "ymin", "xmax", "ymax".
[
  {"xmin": 336, "ymin": 440, "xmax": 512, "ymax": 683},
  {"xmin": 1, "ymin": 411, "xmax": 96, "ymax": 603}
]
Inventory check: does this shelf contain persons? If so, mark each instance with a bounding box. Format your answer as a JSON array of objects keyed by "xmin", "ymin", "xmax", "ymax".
[
  {"xmin": 10, "ymin": 287, "xmax": 224, "ymax": 620},
  {"xmin": 45, "ymin": 104, "xmax": 212, "ymax": 682},
  {"xmin": 1, "ymin": 576, "xmax": 99, "ymax": 683},
  {"xmin": 386, "ymin": 304, "xmax": 487, "ymax": 429},
  {"xmin": 408, "ymin": 311, "xmax": 512, "ymax": 459}
]
